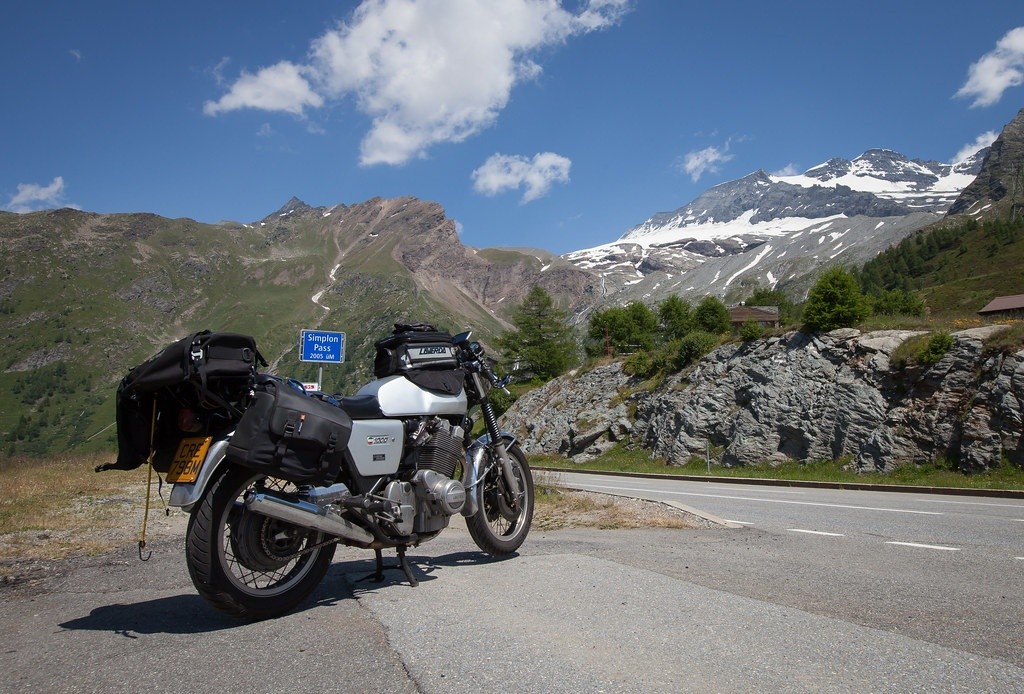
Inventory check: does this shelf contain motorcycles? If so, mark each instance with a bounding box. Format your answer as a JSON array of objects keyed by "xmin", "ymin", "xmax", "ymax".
[{"xmin": 164, "ymin": 324, "xmax": 537, "ymax": 627}]
